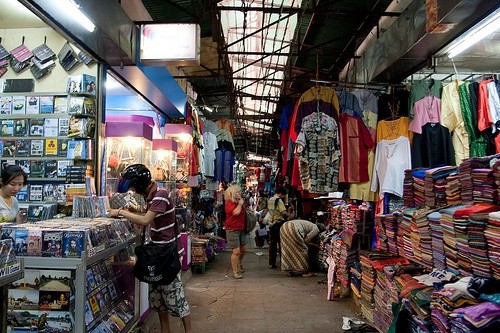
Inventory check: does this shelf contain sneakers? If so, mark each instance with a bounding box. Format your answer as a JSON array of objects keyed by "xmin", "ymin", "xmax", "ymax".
[
  {"xmin": 239, "ymin": 267, "xmax": 245, "ymax": 272},
  {"xmin": 233, "ymin": 272, "xmax": 243, "ymax": 278}
]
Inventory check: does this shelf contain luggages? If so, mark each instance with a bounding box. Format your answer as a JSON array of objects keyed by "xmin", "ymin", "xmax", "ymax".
[{"xmin": 255, "ymin": 230, "xmax": 267, "ymax": 246}]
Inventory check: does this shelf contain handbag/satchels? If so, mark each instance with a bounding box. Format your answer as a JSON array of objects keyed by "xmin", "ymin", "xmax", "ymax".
[
  {"xmin": 269, "ymin": 220, "xmax": 288, "ymax": 242},
  {"xmin": 133, "ymin": 241, "xmax": 181, "ymax": 286},
  {"xmin": 243, "ymin": 211, "xmax": 256, "ymax": 234}
]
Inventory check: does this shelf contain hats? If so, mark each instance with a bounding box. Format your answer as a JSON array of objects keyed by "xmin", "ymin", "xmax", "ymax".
[{"xmin": 117, "ymin": 164, "xmax": 151, "ymax": 193}]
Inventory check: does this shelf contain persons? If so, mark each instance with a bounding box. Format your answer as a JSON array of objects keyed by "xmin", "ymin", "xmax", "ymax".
[
  {"xmin": 191, "ymin": 187, "xmax": 295, "ymax": 249},
  {"xmin": 268, "ymin": 187, "xmax": 290, "ymax": 269},
  {"xmin": 280, "ymin": 219, "xmax": 326, "ymax": 277},
  {"xmin": 224, "ymin": 185, "xmax": 249, "ymax": 280},
  {"xmin": 107, "ymin": 163, "xmax": 193, "ymax": 333},
  {"xmin": 0, "ymin": 165, "xmax": 28, "ymax": 223}
]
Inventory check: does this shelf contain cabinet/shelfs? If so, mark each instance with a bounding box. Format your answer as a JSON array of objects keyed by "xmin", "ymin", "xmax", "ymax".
[{"xmin": 1, "ymin": 92, "xmax": 202, "ymax": 332}]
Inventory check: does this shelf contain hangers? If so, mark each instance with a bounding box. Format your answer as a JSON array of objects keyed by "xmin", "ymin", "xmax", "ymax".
[
  {"xmin": 385, "ymin": 112, "xmax": 401, "ymax": 121},
  {"xmin": 383, "ymin": 126, "xmax": 399, "ymax": 140}
]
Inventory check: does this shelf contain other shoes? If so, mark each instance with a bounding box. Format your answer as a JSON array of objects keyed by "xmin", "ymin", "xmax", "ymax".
[
  {"xmin": 288, "ymin": 271, "xmax": 293, "ymax": 276},
  {"xmin": 302, "ymin": 273, "xmax": 315, "ymax": 277},
  {"xmin": 269, "ymin": 265, "xmax": 277, "ymax": 269}
]
